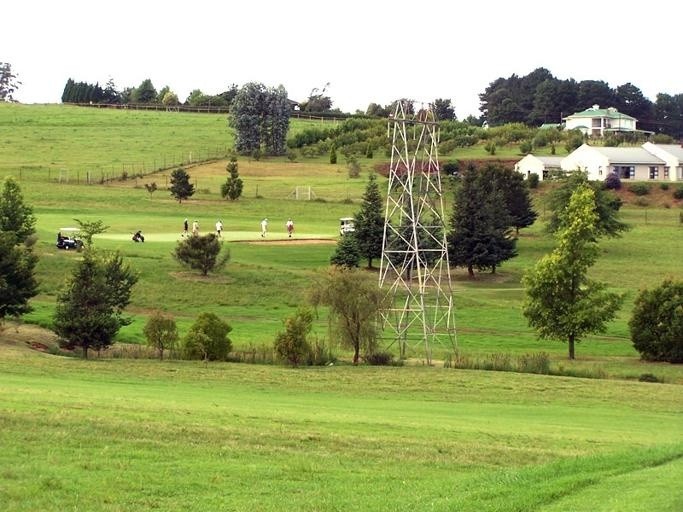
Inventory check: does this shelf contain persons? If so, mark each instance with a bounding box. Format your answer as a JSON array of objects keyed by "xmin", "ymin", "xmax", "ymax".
[
  {"xmin": 132, "ymin": 231, "xmax": 145, "ymax": 242},
  {"xmin": 260, "ymin": 219, "xmax": 268, "ymax": 237},
  {"xmin": 192, "ymin": 220, "xmax": 199, "ymax": 238},
  {"xmin": 215, "ymin": 220, "xmax": 223, "ymax": 238},
  {"xmin": 285, "ymin": 219, "xmax": 293, "ymax": 238},
  {"xmin": 180, "ymin": 218, "xmax": 189, "ymax": 237}
]
